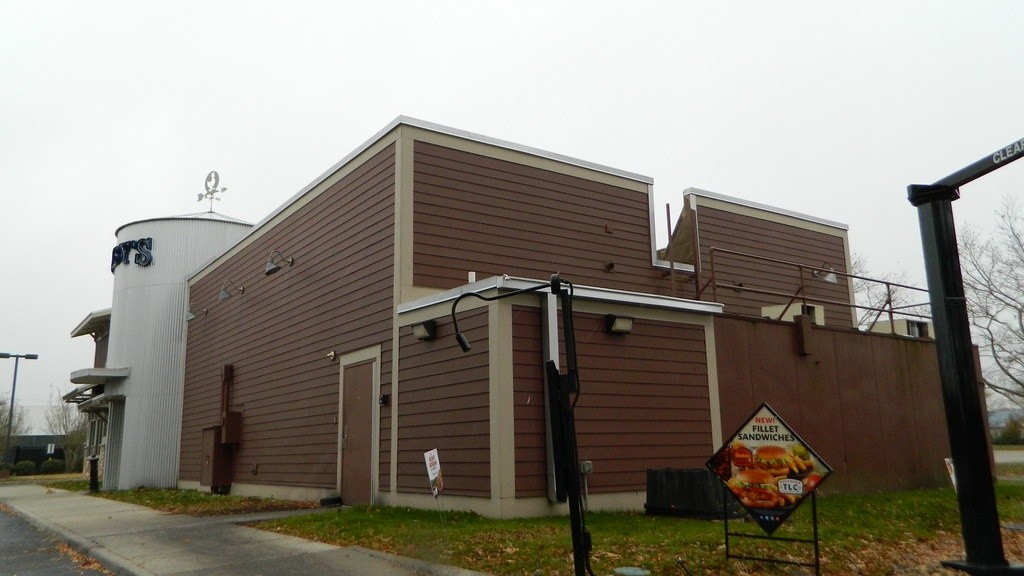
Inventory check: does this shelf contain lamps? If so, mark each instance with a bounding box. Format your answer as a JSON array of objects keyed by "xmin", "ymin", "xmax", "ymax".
[
  {"xmin": 218, "ymin": 280, "xmax": 244, "ymax": 301},
  {"xmin": 185, "ymin": 302, "xmax": 208, "ymax": 321},
  {"xmin": 264, "ymin": 250, "xmax": 293, "ymax": 276},
  {"xmin": 813, "ymin": 262, "xmax": 838, "ymax": 284},
  {"xmin": 602, "ymin": 314, "xmax": 635, "ymax": 333},
  {"xmin": 411, "ymin": 320, "xmax": 437, "ymax": 341}
]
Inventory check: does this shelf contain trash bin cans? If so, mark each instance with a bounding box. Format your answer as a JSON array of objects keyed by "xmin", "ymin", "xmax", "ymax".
[{"xmin": 644, "ymin": 468, "xmax": 741, "ymax": 519}]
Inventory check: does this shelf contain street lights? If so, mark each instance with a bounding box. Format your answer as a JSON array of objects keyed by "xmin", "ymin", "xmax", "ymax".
[{"xmin": 0, "ymin": 351, "xmax": 39, "ymax": 461}]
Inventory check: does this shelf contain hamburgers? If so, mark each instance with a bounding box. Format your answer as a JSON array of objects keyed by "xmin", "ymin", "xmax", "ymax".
[
  {"xmin": 752, "ymin": 445, "xmax": 791, "ymax": 477},
  {"xmin": 726, "ymin": 468, "xmax": 780, "ymax": 507}
]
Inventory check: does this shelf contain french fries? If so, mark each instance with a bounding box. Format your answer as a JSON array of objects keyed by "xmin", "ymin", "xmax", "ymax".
[
  {"xmin": 784, "ymin": 455, "xmax": 813, "ymax": 473},
  {"xmin": 773, "ymin": 487, "xmax": 801, "ymax": 505}
]
